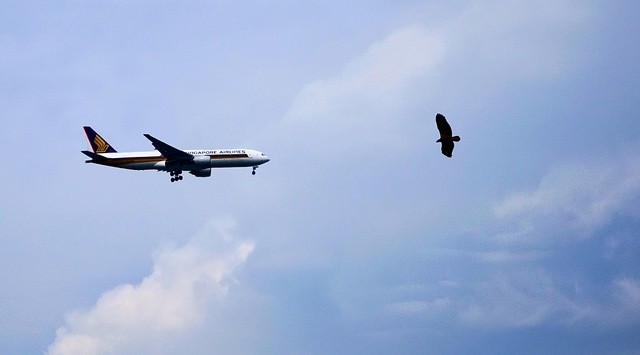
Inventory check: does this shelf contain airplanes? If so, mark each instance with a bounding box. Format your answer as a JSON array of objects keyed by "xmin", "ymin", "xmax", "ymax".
[{"xmin": 81, "ymin": 126, "xmax": 270, "ymax": 183}]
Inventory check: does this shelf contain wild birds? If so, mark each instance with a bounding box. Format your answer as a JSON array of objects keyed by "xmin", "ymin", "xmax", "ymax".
[{"xmin": 435, "ymin": 113, "xmax": 461, "ymax": 158}]
